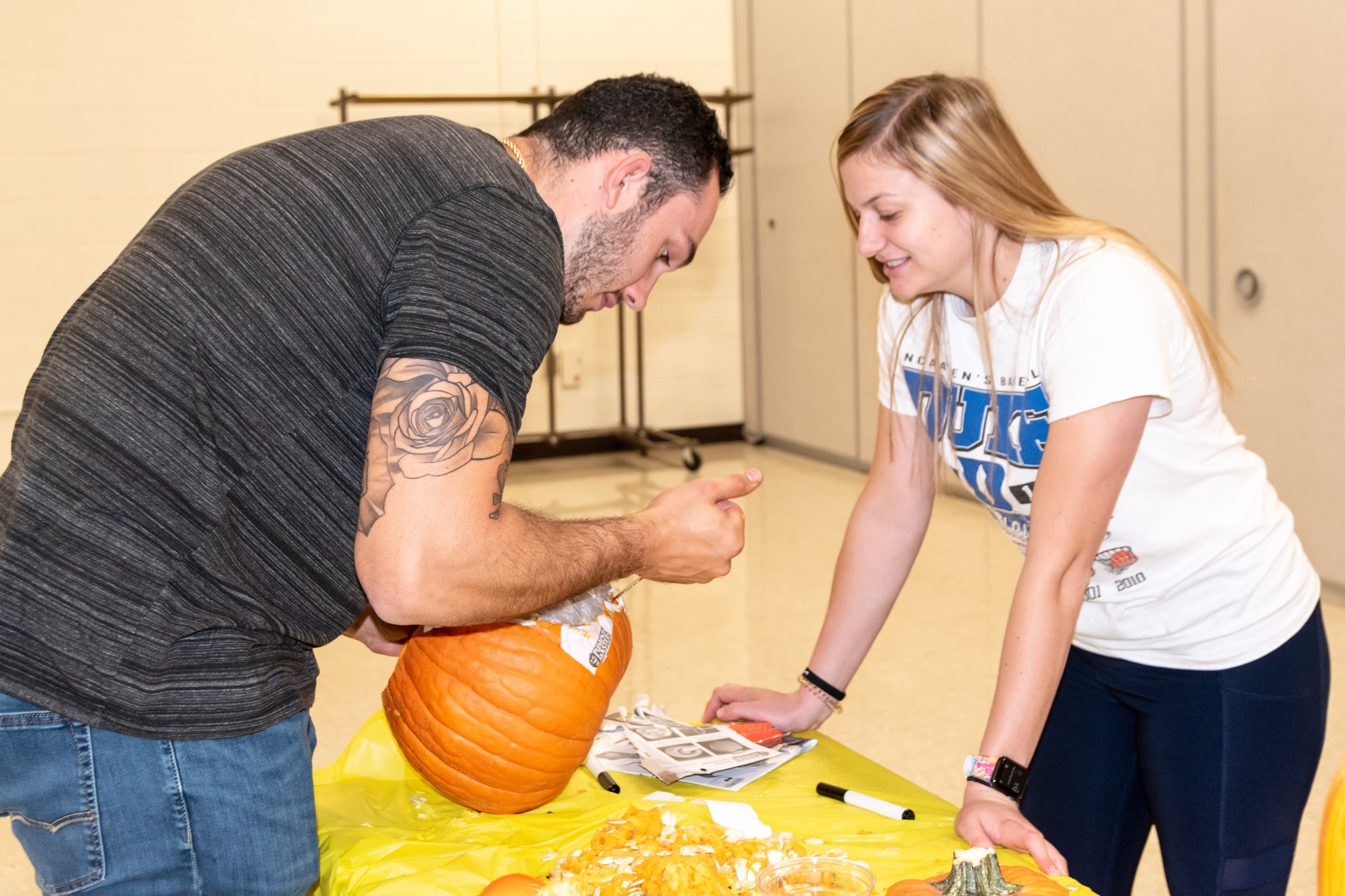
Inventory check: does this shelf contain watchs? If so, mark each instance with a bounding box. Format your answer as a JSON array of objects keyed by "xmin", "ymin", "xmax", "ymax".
[{"xmin": 963, "ymin": 752, "xmax": 1030, "ymax": 802}]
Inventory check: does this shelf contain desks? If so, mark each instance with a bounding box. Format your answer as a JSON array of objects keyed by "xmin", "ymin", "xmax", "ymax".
[{"xmin": 312, "ymin": 719, "xmax": 1100, "ymax": 896}]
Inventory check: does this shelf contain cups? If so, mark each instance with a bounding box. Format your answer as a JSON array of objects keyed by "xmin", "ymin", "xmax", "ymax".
[{"xmin": 757, "ymin": 855, "xmax": 876, "ymax": 896}]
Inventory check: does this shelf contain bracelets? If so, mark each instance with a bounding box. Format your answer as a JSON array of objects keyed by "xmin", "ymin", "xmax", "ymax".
[
  {"xmin": 796, "ymin": 675, "xmax": 843, "ymax": 714},
  {"xmin": 803, "ymin": 667, "xmax": 846, "ymax": 701}
]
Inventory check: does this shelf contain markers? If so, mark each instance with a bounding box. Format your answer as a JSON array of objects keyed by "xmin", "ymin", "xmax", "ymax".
[
  {"xmin": 816, "ymin": 782, "xmax": 915, "ymax": 820},
  {"xmin": 583, "ymin": 751, "xmax": 620, "ymax": 794}
]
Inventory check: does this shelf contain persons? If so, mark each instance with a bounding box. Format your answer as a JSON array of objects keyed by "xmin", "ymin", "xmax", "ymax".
[
  {"xmin": 702, "ymin": 75, "xmax": 1333, "ymax": 896},
  {"xmin": 0, "ymin": 74, "xmax": 734, "ymax": 896}
]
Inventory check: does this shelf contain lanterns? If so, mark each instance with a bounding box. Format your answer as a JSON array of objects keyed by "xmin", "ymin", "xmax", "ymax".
[{"xmin": 380, "ymin": 577, "xmax": 634, "ymax": 817}]
